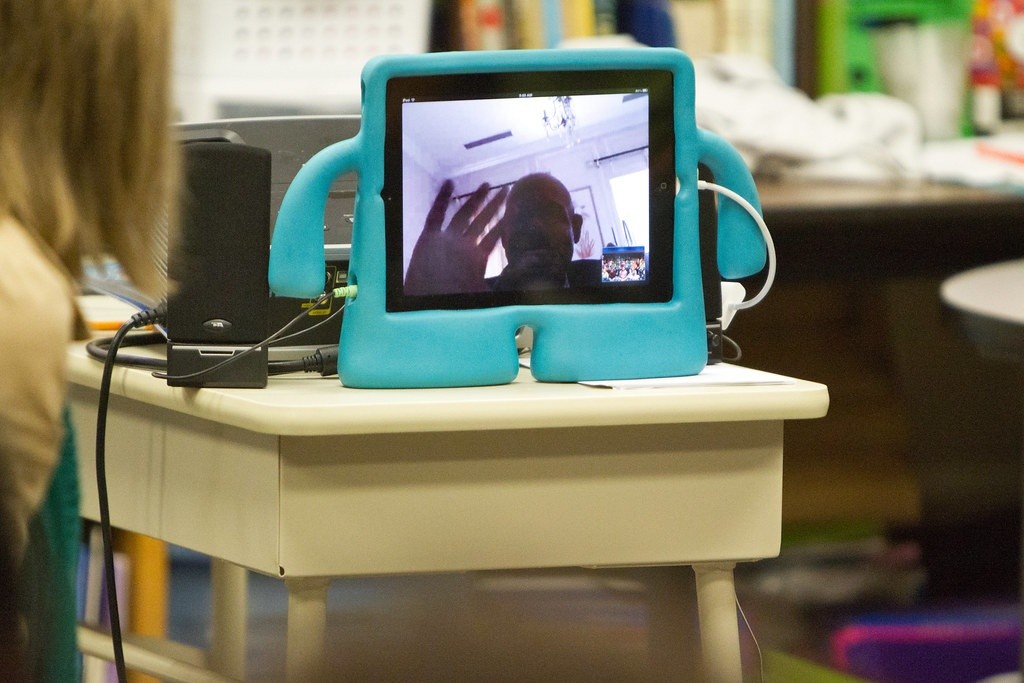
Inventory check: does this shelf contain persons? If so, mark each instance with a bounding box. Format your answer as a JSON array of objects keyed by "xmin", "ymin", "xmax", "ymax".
[
  {"xmin": 400, "ymin": 173, "xmax": 606, "ymax": 299},
  {"xmin": 0, "ymin": 2, "xmax": 179, "ymax": 683}
]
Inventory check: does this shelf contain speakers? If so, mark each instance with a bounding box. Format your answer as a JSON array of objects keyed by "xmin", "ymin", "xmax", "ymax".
[{"xmin": 165, "ymin": 141, "xmax": 272, "ymax": 388}]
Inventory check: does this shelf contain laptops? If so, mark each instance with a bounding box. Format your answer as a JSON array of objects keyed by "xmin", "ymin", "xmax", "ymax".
[{"xmin": 147, "ymin": 113, "xmax": 363, "ymax": 361}]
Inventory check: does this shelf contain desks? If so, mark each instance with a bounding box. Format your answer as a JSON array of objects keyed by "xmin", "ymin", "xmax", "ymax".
[
  {"xmin": 65, "ymin": 326, "xmax": 829, "ymax": 683},
  {"xmin": 754, "ymin": 172, "xmax": 1023, "ymax": 510}
]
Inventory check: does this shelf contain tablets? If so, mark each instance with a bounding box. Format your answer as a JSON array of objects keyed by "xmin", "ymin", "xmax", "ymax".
[{"xmin": 383, "ymin": 70, "xmax": 675, "ymax": 314}]
